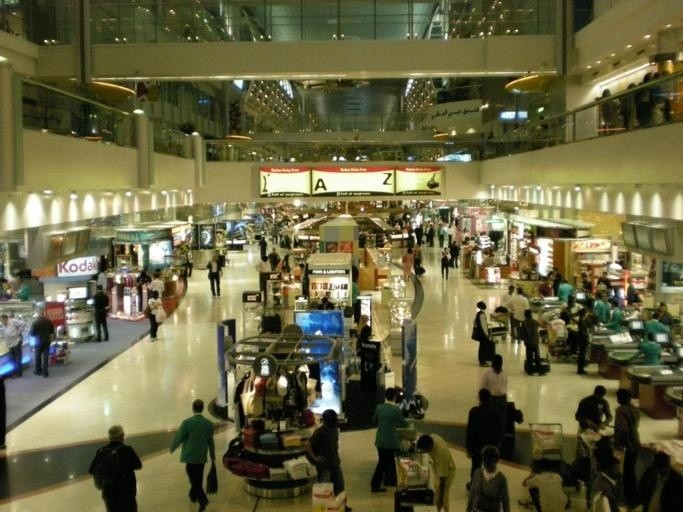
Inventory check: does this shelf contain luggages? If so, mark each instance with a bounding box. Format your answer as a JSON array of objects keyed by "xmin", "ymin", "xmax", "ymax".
[{"xmin": 480, "ymin": 324, "xmax": 496, "ymax": 361}]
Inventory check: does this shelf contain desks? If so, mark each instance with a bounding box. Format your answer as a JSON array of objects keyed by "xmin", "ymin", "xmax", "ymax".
[{"xmin": 648, "ymin": 440, "xmax": 682, "ymax": 476}]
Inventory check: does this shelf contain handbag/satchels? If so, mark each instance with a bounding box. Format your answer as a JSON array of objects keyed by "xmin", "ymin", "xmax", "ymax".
[
  {"xmin": 207, "ymin": 463, "xmax": 217, "ymax": 493},
  {"xmin": 144, "ymin": 304, "xmax": 151, "ymax": 318},
  {"xmin": 312, "ymin": 484, "xmax": 334, "ymax": 512},
  {"xmin": 235, "ymin": 372, "xmax": 265, "ymax": 417},
  {"xmin": 326, "ymin": 491, "xmax": 347, "ymax": 512},
  {"xmin": 284, "ymin": 374, "xmax": 303, "ymax": 409},
  {"xmin": 472, "ymin": 326, "xmax": 484, "ymax": 341}
]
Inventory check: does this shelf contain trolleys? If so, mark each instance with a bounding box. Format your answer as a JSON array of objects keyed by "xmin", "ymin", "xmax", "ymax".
[
  {"xmin": 539, "ymin": 329, "xmax": 548, "ymax": 345},
  {"xmin": 393, "ymin": 452, "xmax": 432, "ymax": 498},
  {"xmin": 576, "ymin": 423, "xmax": 620, "ymax": 482},
  {"xmin": 544, "ymin": 320, "xmax": 570, "ymax": 358},
  {"xmin": 521, "ymin": 420, "xmax": 564, "ymax": 478},
  {"xmin": 47, "ymin": 336, "xmax": 73, "ymax": 366},
  {"xmin": 522, "ymin": 349, "xmax": 553, "ymax": 373},
  {"xmin": 486, "ymin": 312, "xmax": 508, "ymax": 342}
]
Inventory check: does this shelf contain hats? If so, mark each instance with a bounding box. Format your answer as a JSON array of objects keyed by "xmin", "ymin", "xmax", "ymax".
[
  {"xmin": 277, "ymin": 378, "xmax": 288, "ymax": 396},
  {"xmin": 266, "ymin": 376, "xmax": 277, "ymax": 395}
]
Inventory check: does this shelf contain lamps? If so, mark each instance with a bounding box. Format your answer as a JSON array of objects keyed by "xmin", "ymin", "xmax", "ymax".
[
  {"xmin": 181, "ymin": 122, "xmax": 198, "ymax": 138},
  {"xmin": 131, "ymin": 97, "xmax": 146, "ymax": 115}
]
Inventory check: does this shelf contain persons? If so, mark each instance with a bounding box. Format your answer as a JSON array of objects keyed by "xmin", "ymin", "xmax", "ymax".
[
  {"xmin": 88, "ymin": 245, "xmax": 223, "ymax": 343},
  {"xmin": 474, "ymin": 115, "xmax": 554, "ymax": 162},
  {"xmin": 255, "ymin": 214, "xmax": 292, "ymax": 272},
  {"xmin": 1, "ymin": 382, "xmax": 8, "ymax": 450},
  {"xmin": 595, "ymin": 71, "xmax": 672, "ymax": 137},
  {"xmin": 293, "ymin": 202, "xmax": 683, "ymax": 512},
  {"xmin": 181, "ymin": 123, "xmax": 238, "ymax": 161},
  {"xmin": 79, "ymin": 94, "xmax": 104, "ymax": 137},
  {"xmin": 343, "ymin": 145, "xmax": 384, "ymax": 161},
  {"xmin": 89, "ymin": 399, "xmax": 216, "ymax": 512},
  {"xmin": 0, "ymin": 277, "xmax": 56, "ymax": 379}
]
